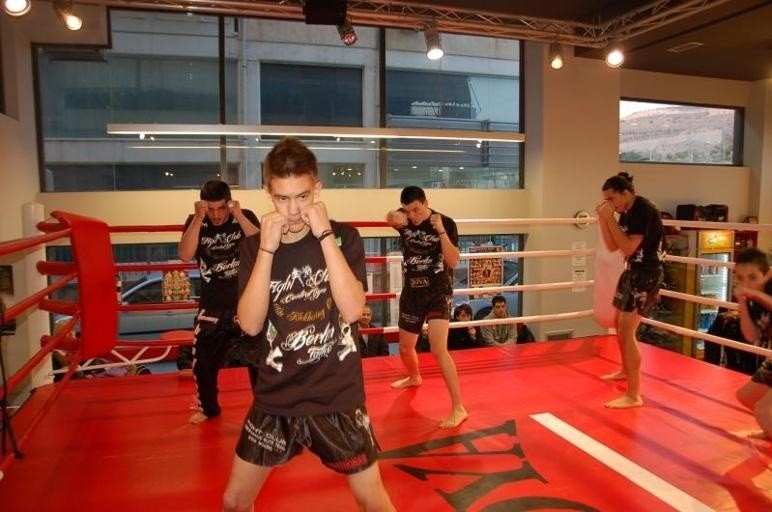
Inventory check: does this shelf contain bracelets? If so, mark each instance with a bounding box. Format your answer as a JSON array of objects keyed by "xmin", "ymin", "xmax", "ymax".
[
  {"xmin": 258, "ymin": 247, "xmax": 276, "ymax": 255},
  {"xmin": 439, "ymin": 232, "xmax": 447, "ymax": 235},
  {"xmin": 316, "ymin": 228, "xmax": 334, "ymax": 243}
]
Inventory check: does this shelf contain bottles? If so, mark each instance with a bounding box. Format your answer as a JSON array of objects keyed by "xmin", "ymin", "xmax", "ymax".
[{"xmin": 698, "ymin": 313, "xmax": 717, "ymax": 329}]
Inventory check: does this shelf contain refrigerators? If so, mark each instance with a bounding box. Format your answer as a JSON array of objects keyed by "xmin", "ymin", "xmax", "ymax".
[{"xmin": 655, "ymin": 225, "xmax": 729, "ymax": 365}]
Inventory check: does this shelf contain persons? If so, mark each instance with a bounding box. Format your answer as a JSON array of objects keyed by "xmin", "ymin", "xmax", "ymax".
[
  {"xmin": 387, "ymin": 185, "xmax": 469, "ymax": 430},
  {"xmin": 222, "ymin": 135, "xmax": 399, "ymax": 512},
  {"xmin": 357, "ymin": 306, "xmax": 389, "ymax": 358},
  {"xmin": 447, "ymin": 303, "xmax": 485, "ymax": 351},
  {"xmin": 595, "ymin": 171, "xmax": 663, "ymax": 408},
  {"xmin": 733, "ymin": 248, "xmax": 772, "ymax": 440},
  {"xmin": 481, "ymin": 295, "xmax": 519, "ymax": 345},
  {"xmin": 178, "ymin": 180, "xmax": 261, "ymax": 424}
]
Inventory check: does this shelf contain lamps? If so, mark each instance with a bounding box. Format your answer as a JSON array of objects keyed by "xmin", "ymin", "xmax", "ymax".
[
  {"xmin": 333, "ymin": 15, "xmax": 359, "ymax": 48},
  {"xmin": 425, "ymin": 22, "xmax": 445, "ymax": 62},
  {"xmin": 547, "ymin": 38, "xmax": 628, "ymax": 72},
  {"xmin": 54, "ymin": 1, "xmax": 85, "ymax": 33}
]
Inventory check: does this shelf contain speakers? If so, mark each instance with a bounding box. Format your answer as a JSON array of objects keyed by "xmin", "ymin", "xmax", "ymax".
[{"xmin": 303, "ymin": 0, "xmax": 346, "ymax": 25}]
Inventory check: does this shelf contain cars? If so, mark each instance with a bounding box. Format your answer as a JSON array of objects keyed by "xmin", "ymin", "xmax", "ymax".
[
  {"xmin": 55, "ymin": 267, "xmax": 202, "ymax": 344},
  {"xmin": 450, "ymin": 260, "xmax": 521, "ymax": 318}
]
